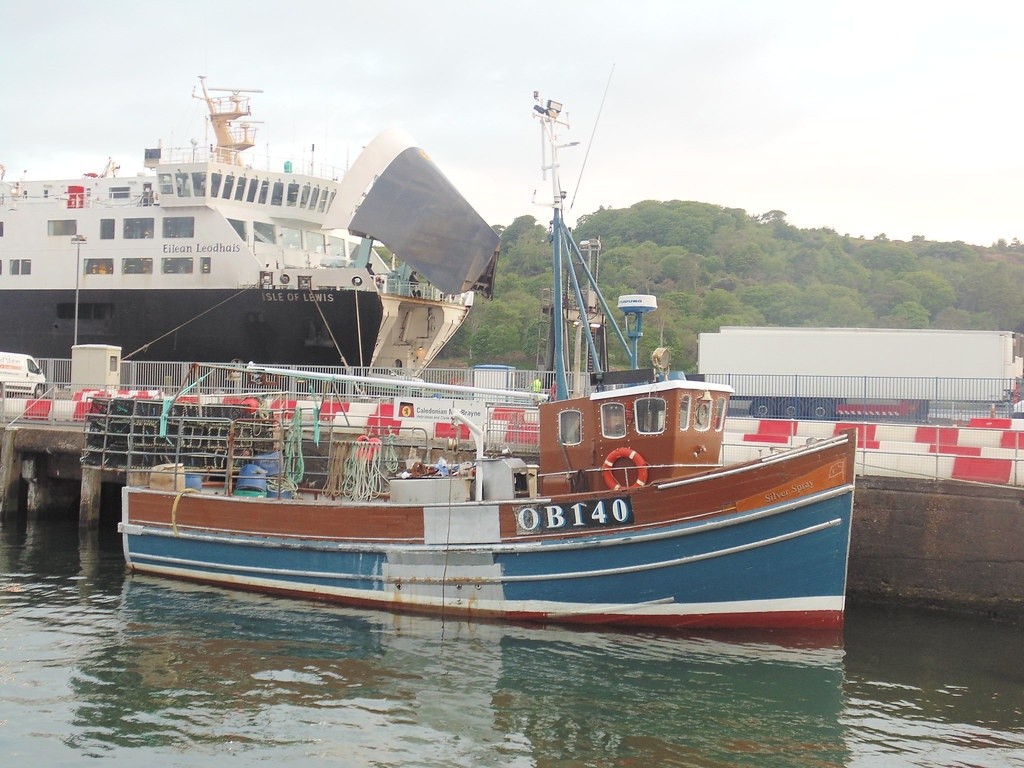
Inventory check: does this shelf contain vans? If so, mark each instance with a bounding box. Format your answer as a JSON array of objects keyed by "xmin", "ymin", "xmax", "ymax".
[{"xmin": 0, "ymin": 351, "xmax": 47, "ymax": 399}]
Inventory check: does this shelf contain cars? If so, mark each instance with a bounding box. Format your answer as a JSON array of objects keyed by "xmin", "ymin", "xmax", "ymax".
[{"xmin": 367, "ymin": 367, "xmax": 426, "ymax": 383}]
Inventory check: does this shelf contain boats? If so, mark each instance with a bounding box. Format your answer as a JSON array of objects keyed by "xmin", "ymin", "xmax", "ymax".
[
  {"xmin": 0, "ymin": 73, "xmax": 503, "ymax": 394},
  {"xmin": 114, "ymin": 62, "xmax": 861, "ymax": 630}
]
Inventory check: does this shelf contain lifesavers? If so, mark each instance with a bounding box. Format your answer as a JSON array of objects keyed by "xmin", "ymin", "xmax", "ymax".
[{"xmin": 599, "ymin": 446, "xmax": 651, "ymax": 493}]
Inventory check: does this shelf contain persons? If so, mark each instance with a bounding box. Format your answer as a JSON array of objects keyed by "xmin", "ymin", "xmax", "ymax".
[
  {"xmin": 531, "ymin": 376, "xmax": 542, "ymax": 394},
  {"xmin": 550, "ymin": 381, "xmax": 558, "ymax": 398},
  {"xmin": 408, "ymin": 270, "xmax": 421, "ymax": 297}
]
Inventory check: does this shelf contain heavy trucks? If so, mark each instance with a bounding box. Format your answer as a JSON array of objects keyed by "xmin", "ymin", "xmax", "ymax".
[{"xmin": 696, "ymin": 325, "xmax": 1024, "ymax": 421}]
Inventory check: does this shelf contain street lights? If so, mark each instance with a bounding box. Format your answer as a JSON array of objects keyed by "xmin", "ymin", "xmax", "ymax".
[{"xmin": 71, "ymin": 234, "xmax": 87, "ymax": 345}]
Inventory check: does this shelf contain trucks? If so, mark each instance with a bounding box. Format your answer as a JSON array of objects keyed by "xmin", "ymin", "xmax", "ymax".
[{"xmin": 471, "ymin": 363, "xmax": 516, "ymax": 405}]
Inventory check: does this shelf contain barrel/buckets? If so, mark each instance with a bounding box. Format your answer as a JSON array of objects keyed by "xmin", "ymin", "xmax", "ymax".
[
  {"xmin": 185, "ymin": 473, "xmax": 203, "ymax": 493},
  {"xmin": 236, "ymin": 464, "xmax": 267, "ymax": 491}
]
